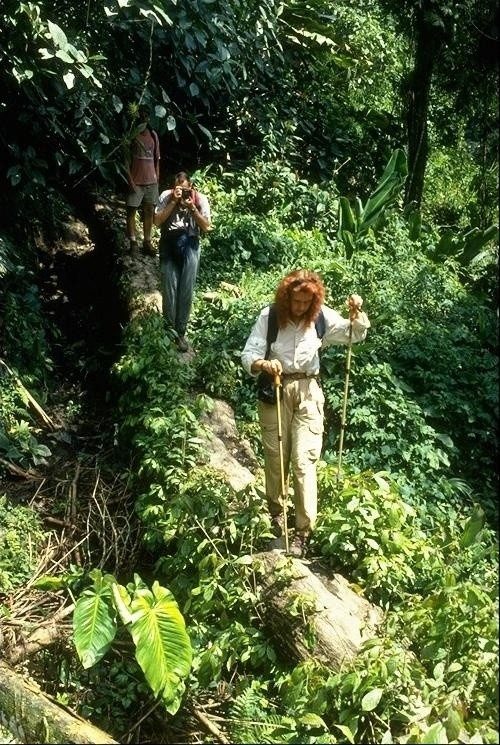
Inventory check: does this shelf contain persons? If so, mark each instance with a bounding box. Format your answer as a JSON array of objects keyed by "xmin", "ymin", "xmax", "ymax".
[
  {"xmin": 241, "ymin": 270, "xmax": 370, "ymax": 558},
  {"xmin": 152, "ymin": 172, "xmax": 212, "ymax": 351},
  {"xmin": 127, "ymin": 105, "xmax": 161, "ymax": 256}
]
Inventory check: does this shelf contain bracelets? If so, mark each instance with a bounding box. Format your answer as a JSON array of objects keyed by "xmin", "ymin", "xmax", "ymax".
[
  {"xmin": 190, "ymin": 207, "xmax": 197, "ymax": 214},
  {"xmin": 261, "ymin": 361, "xmax": 266, "ymax": 370}
]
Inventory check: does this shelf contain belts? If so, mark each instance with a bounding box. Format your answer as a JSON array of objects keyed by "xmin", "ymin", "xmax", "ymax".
[{"xmin": 283, "ymin": 373, "xmax": 317, "ymax": 379}]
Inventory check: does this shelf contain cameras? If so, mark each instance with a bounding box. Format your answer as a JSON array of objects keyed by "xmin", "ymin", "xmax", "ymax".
[{"xmin": 177, "ymin": 188, "xmax": 191, "ymax": 199}]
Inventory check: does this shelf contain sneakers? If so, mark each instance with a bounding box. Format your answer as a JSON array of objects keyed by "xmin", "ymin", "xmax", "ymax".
[
  {"xmin": 175, "ymin": 337, "xmax": 190, "ymax": 353},
  {"xmin": 289, "ymin": 532, "xmax": 312, "ymax": 557},
  {"xmin": 128, "ymin": 241, "xmax": 137, "ymax": 257},
  {"xmin": 271, "ymin": 514, "xmax": 284, "ymax": 537},
  {"xmin": 142, "ymin": 240, "xmax": 158, "ymax": 256}
]
